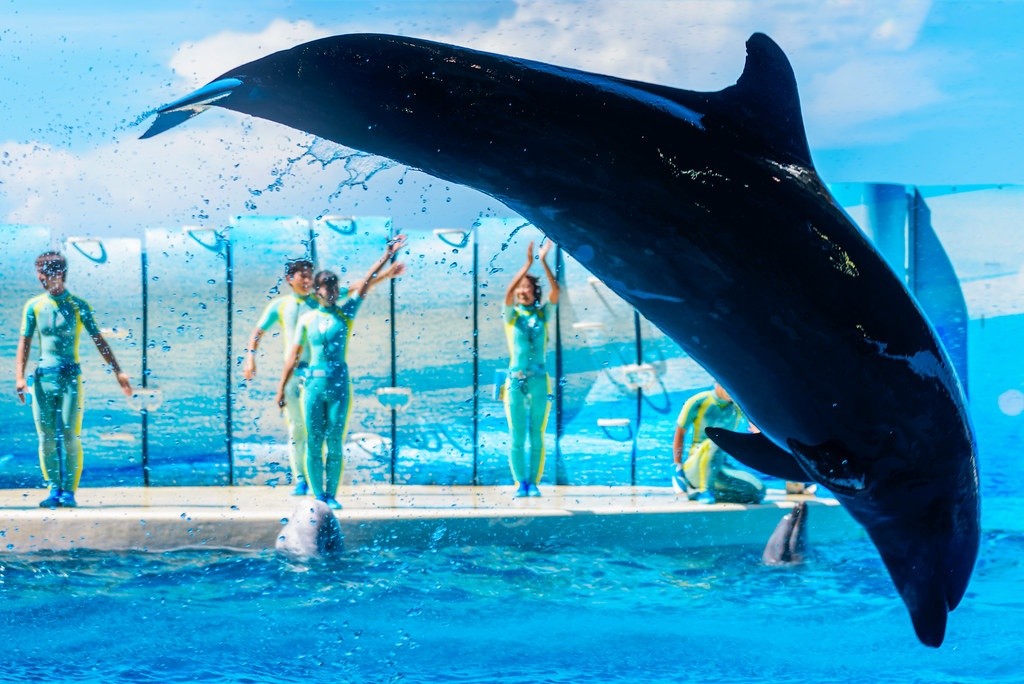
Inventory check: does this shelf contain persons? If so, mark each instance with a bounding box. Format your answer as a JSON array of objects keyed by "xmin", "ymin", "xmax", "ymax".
[
  {"xmin": 672, "ymin": 381, "xmax": 767, "ymax": 506},
  {"xmin": 276, "ymin": 231, "xmax": 410, "ymax": 508},
  {"xmin": 16, "ymin": 251, "xmax": 133, "ymax": 508},
  {"xmin": 502, "ymin": 239, "xmax": 561, "ymax": 498},
  {"xmin": 242, "ymin": 262, "xmax": 404, "ymax": 496}
]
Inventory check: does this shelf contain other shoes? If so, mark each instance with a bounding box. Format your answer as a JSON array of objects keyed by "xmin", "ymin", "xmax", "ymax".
[
  {"xmin": 40, "ymin": 494, "xmax": 61, "ymax": 508},
  {"xmin": 316, "ymin": 495, "xmax": 344, "ymax": 511},
  {"xmin": 514, "ymin": 482, "xmax": 542, "ymax": 497},
  {"xmin": 696, "ymin": 491, "xmax": 716, "ymax": 506},
  {"xmin": 291, "ymin": 480, "xmax": 308, "ymax": 497},
  {"xmin": 59, "ymin": 492, "xmax": 77, "ymax": 508}
]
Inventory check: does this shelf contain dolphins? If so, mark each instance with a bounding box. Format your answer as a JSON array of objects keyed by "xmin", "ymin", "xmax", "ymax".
[{"xmin": 139, "ymin": 32, "xmax": 982, "ymax": 649}]
